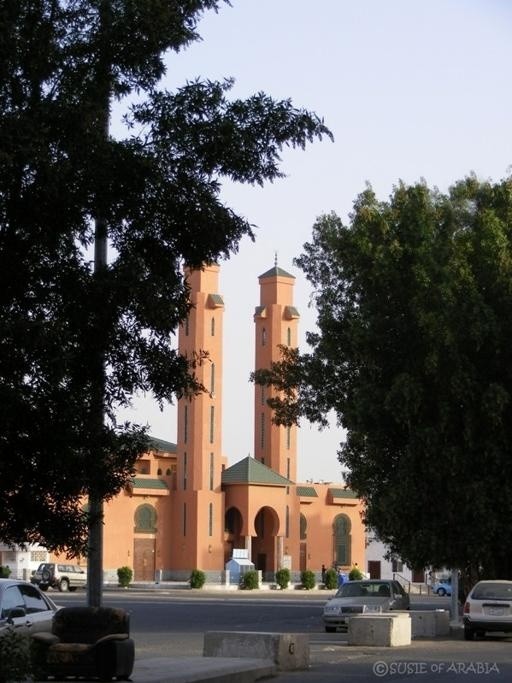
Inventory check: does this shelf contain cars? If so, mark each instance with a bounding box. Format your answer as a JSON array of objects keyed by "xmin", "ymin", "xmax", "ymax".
[
  {"xmin": 432, "ymin": 577, "xmax": 452, "ymax": 596},
  {"xmin": 463, "ymin": 580, "xmax": 512, "ymax": 639},
  {"xmin": 322, "ymin": 579, "xmax": 409, "ymax": 633},
  {"xmin": 0, "ymin": 578, "xmax": 66, "ymax": 658}
]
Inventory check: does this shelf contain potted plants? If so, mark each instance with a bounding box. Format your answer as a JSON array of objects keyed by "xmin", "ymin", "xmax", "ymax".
[
  {"xmin": 190, "ymin": 564, "xmax": 335, "ymax": 594},
  {"xmin": 116, "ymin": 566, "xmax": 133, "ymax": 590}
]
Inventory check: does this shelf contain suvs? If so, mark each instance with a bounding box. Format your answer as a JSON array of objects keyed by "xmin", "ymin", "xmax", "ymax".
[{"xmin": 30, "ymin": 562, "xmax": 88, "ymax": 591}]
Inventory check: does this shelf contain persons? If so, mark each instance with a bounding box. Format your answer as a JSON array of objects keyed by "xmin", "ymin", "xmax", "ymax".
[
  {"xmin": 322, "ymin": 565, "xmax": 327, "ymax": 583},
  {"xmin": 353, "ymin": 562, "xmax": 358, "ymax": 569}
]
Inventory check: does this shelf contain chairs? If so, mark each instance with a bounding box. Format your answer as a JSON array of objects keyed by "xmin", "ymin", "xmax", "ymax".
[
  {"xmin": 28, "ymin": 605, "xmax": 135, "ymax": 682},
  {"xmin": 349, "ymin": 584, "xmax": 389, "ymax": 594}
]
